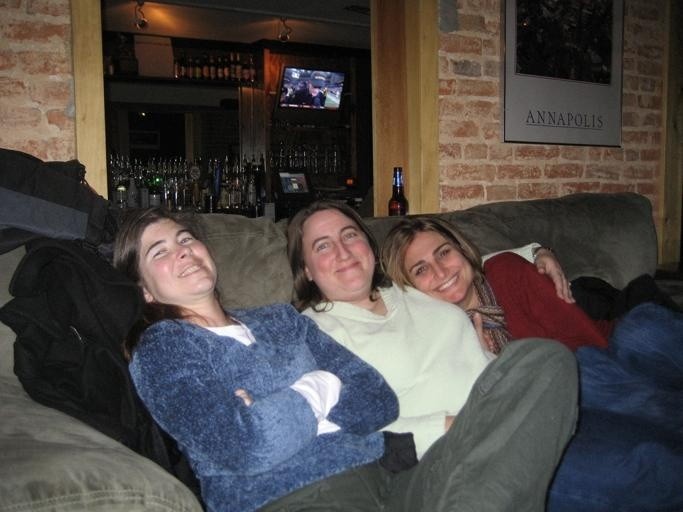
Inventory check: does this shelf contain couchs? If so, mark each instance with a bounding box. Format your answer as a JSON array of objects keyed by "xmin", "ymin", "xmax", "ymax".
[{"xmin": 0, "ymin": 190, "xmax": 680, "ymax": 510}]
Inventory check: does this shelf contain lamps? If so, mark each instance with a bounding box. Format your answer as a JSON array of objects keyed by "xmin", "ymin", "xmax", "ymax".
[
  {"xmin": 134, "ymin": 1, "xmax": 149, "ymax": 31},
  {"xmin": 276, "ymin": 15, "xmax": 295, "ymax": 43}
]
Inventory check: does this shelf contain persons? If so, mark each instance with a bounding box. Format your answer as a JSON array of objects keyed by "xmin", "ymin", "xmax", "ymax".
[
  {"xmin": 282, "ymin": 199, "xmax": 577, "ymax": 461},
  {"xmin": 111, "ymin": 203, "xmax": 581, "ymax": 512},
  {"xmin": 378, "ymin": 213, "xmax": 614, "ymax": 359},
  {"xmin": 279, "ymin": 67, "xmax": 342, "ymax": 108}
]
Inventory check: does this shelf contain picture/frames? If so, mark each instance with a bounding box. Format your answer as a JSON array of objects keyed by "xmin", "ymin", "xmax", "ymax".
[{"xmin": 500, "ymin": 1, "xmax": 625, "ymax": 150}]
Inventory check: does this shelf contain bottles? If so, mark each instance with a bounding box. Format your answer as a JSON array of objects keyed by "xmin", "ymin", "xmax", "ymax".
[
  {"xmin": 107, "ymin": 143, "xmax": 338, "ymax": 211},
  {"xmin": 388, "ymin": 166, "xmax": 408, "ymax": 217},
  {"xmin": 172, "ymin": 51, "xmax": 257, "ymax": 83}
]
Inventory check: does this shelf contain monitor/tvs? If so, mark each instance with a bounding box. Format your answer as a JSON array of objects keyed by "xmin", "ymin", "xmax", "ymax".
[
  {"xmin": 277, "ymin": 170, "xmax": 312, "ymax": 194},
  {"xmin": 276, "ymin": 66, "xmax": 348, "ymax": 113}
]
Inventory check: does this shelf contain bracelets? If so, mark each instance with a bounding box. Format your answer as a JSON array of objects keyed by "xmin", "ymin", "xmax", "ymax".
[{"xmin": 533, "ymin": 246, "xmax": 554, "ymax": 257}]
